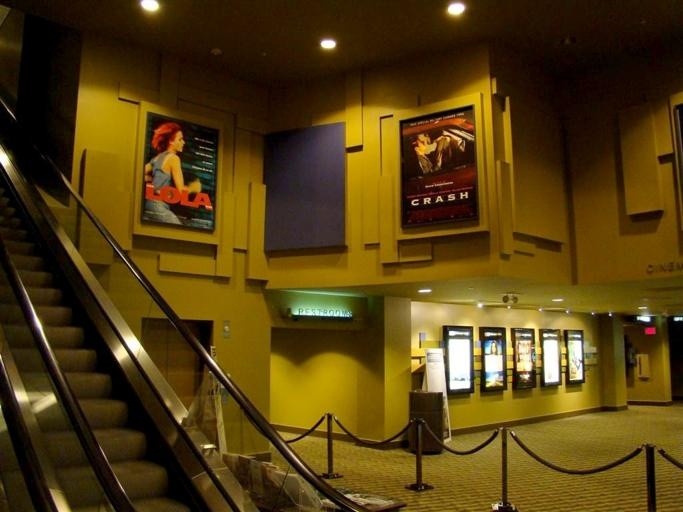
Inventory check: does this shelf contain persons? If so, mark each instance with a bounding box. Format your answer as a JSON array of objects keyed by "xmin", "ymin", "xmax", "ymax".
[
  {"xmin": 491, "ymin": 341, "xmax": 497, "ymax": 355},
  {"xmin": 143, "ymin": 121, "xmax": 202, "ymax": 225}
]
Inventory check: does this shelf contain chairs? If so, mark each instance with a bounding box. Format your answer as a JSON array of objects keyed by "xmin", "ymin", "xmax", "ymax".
[{"xmin": 222, "ymin": 452, "xmax": 342, "ymax": 512}]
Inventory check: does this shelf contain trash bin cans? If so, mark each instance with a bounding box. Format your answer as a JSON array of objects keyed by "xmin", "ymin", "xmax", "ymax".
[{"xmin": 408, "ymin": 389, "xmax": 444, "ymax": 456}]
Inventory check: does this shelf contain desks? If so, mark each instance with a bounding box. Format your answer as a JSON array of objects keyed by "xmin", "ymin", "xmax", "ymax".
[{"xmin": 319, "ymin": 490, "xmax": 408, "ymax": 511}]
